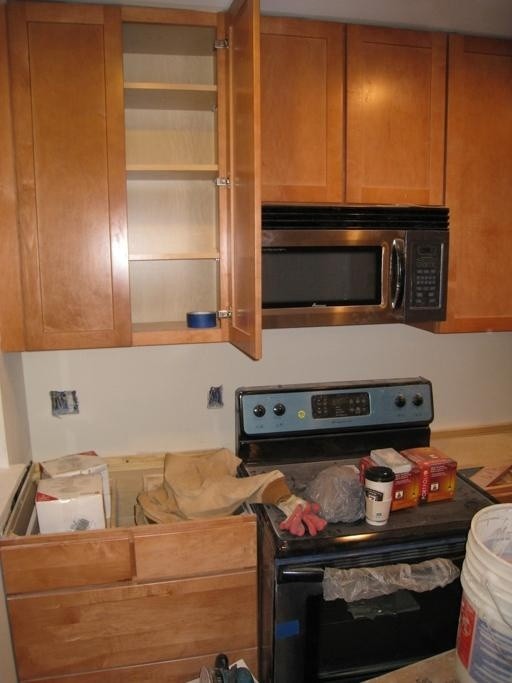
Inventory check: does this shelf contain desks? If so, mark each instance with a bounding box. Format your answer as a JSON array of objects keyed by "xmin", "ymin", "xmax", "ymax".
[{"xmin": 358, "ymin": 649, "xmax": 458, "ymax": 683}]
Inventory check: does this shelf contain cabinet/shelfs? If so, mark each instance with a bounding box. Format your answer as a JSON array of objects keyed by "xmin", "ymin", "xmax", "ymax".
[
  {"xmin": 257, "ymin": 14, "xmax": 446, "ymax": 207},
  {"xmin": 437, "ymin": 32, "xmax": 511, "ymax": 336},
  {"xmin": 2, "ymin": 2, "xmax": 261, "ymax": 359},
  {"xmin": 0, "ymin": 449, "xmax": 258, "ymax": 682}
]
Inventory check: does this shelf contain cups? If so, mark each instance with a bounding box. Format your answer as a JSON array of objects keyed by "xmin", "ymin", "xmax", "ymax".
[{"xmin": 363, "ymin": 466, "xmax": 396, "ymax": 528}]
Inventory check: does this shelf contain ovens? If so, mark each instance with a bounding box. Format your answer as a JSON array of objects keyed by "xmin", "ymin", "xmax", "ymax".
[{"xmin": 235, "ymin": 376, "xmax": 502, "ymax": 683}]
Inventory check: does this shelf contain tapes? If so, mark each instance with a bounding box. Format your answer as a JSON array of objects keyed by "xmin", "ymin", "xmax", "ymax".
[{"xmin": 187, "ymin": 311, "xmax": 216, "ymax": 327}]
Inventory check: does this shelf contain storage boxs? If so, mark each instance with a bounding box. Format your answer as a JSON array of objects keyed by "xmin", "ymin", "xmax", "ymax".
[
  {"xmin": 35, "ymin": 451, "xmax": 112, "ymax": 534},
  {"xmin": 360, "ymin": 447, "xmax": 457, "ymax": 512}
]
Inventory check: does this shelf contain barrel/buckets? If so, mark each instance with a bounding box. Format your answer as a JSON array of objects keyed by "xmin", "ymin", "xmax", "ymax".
[{"xmin": 454, "ymin": 503, "xmax": 512, "ymax": 683}]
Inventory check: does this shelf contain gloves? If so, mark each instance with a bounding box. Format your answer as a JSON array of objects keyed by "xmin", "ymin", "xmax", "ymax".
[{"xmin": 277, "ymin": 493, "xmax": 327, "ymax": 537}]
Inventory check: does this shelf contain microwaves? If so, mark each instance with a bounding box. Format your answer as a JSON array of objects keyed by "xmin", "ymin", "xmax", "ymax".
[{"xmin": 261, "ymin": 228, "xmax": 450, "ymax": 330}]
